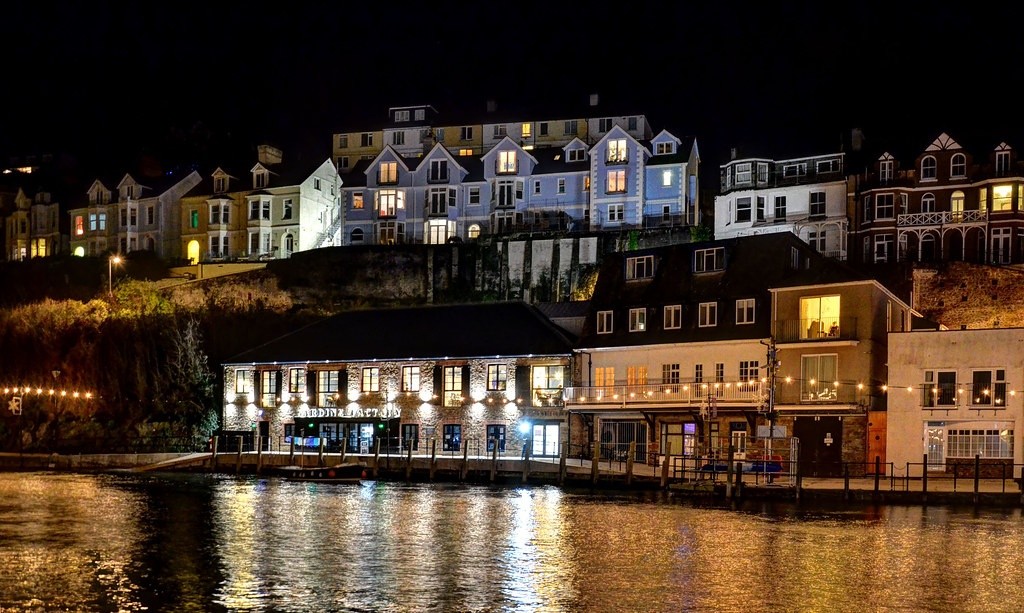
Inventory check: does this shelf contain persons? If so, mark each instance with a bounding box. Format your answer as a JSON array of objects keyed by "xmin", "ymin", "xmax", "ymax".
[
  {"xmin": 536, "ymin": 385, "xmax": 542, "ymax": 398},
  {"xmin": 554, "ymin": 384, "xmax": 562, "ymax": 403},
  {"xmin": 829, "ymin": 321, "xmax": 838, "ymax": 336}
]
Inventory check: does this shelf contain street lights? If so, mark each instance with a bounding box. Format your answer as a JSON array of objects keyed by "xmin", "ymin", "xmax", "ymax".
[{"xmin": 108, "ymin": 256, "xmax": 121, "ymax": 298}]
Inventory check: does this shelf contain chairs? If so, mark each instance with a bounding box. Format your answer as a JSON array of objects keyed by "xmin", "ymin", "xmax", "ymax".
[{"xmin": 807, "ymin": 318, "xmax": 824, "ymax": 338}]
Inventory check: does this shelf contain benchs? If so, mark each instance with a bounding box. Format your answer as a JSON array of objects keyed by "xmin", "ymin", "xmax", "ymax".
[
  {"xmin": 672, "ymin": 457, "xmax": 719, "ymax": 483},
  {"xmin": 703, "ymin": 463, "xmax": 781, "ymax": 483}
]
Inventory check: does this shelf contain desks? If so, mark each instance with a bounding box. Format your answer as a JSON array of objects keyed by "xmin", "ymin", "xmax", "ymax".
[{"xmin": 817, "ymin": 330, "xmax": 827, "ymax": 337}]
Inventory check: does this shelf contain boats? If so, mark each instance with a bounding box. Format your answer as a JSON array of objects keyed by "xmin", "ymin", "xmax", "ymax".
[{"xmin": 278, "ymin": 463, "xmax": 365, "ymax": 484}]
